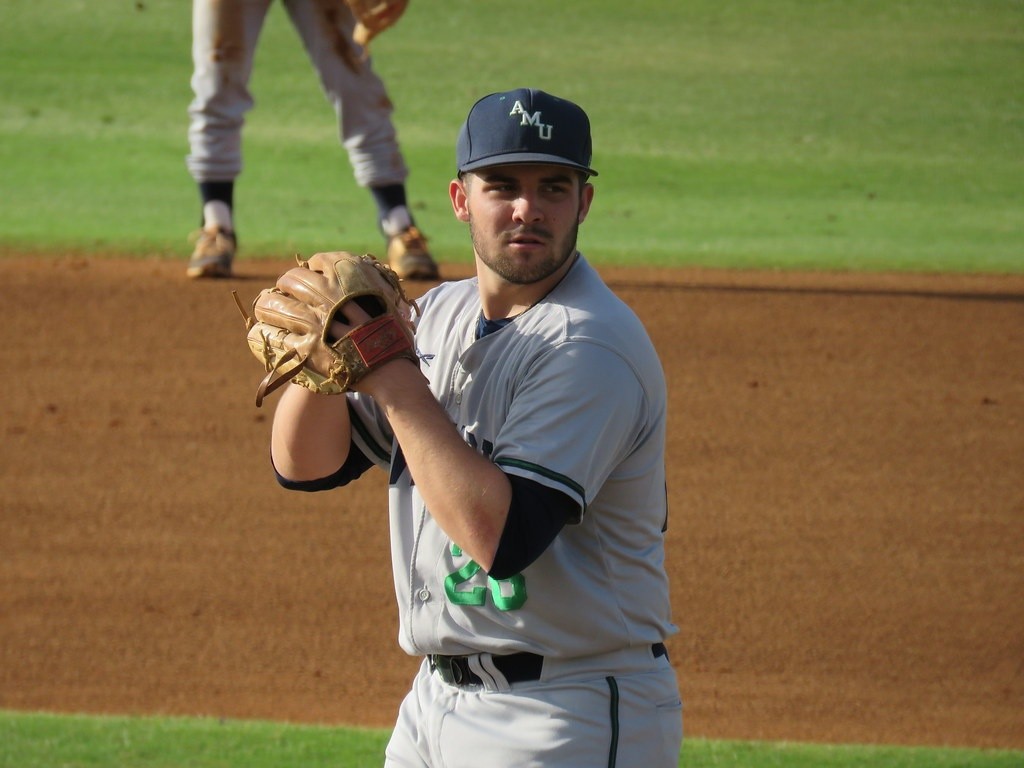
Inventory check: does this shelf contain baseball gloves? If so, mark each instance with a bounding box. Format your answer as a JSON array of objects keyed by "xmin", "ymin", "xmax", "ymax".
[
  {"xmin": 227, "ymin": 249, "xmax": 422, "ymax": 410},
  {"xmin": 345, "ymin": 0, "xmax": 410, "ymax": 47}
]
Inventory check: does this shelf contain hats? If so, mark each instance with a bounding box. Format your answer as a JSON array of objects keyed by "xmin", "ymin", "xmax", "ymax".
[{"xmin": 457, "ymin": 88, "xmax": 598, "ymax": 182}]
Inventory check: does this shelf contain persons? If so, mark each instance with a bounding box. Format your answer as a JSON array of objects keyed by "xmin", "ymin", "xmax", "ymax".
[
  {"xmin": 186, "ymin": 1, "xmax": 445, "ymax": 277},
  {"xmin": 273, "ymin": 88, "xmax": 684, "ymax": 768}
]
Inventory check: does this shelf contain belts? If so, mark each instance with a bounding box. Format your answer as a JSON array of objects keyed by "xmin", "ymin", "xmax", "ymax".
[{"xmin": 434, "ymin": 643, "xmax": 668, "ymax": 684}]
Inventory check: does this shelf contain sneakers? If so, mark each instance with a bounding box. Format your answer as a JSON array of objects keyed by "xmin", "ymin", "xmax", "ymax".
[
  {"xmin": 187, "ymin": 225, "xmax": 236, "ymax": 277},
  {"xmin": 385, "ymin": 226, "xmax": 440, "ymax": 279}
]
